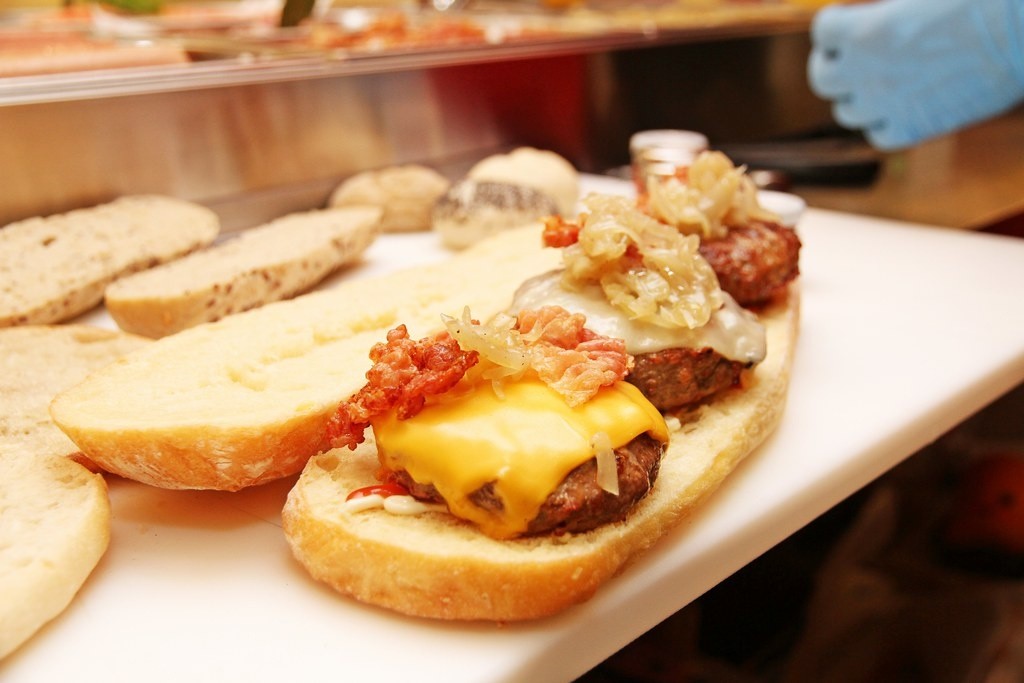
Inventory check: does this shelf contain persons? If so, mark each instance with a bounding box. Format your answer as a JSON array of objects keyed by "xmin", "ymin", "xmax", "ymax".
[{"xmin": 807, "ymin": 0, "xmax": 1024, "ymax": 153}]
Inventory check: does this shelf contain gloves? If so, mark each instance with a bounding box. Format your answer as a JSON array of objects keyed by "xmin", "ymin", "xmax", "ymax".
[{"xmin": 808, "ymin": 0, "xmax": 1024, "ymax": 153}]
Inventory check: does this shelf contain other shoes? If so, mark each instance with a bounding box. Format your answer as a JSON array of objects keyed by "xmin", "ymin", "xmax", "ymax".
[{"xmin": 935, "ymin": 452, "xmax": 1024, "ymax": 576}]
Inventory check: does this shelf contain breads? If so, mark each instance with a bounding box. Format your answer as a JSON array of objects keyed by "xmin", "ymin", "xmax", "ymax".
[{"xmin": 1, "ymin": 147, "xmax": 803, "ymax": 667}]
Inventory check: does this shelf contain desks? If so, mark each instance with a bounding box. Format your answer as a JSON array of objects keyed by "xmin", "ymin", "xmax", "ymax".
[{"xmin": 0, "ymin": 167, "xmax": 1024, "ymax": 683}]
